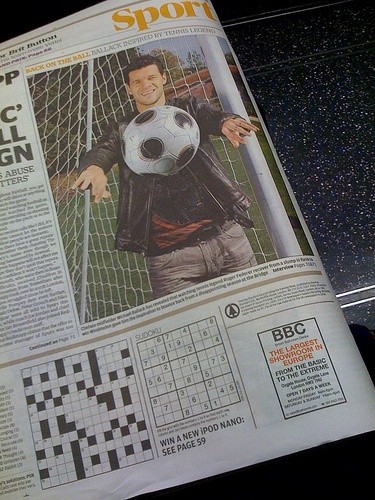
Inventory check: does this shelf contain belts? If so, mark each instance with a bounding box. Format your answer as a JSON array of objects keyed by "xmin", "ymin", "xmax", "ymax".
[{"xmin": 150, "ymin": 218, "xmax": 227, "ymax": 257}]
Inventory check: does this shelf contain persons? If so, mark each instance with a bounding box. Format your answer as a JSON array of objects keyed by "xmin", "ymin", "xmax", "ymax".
[{"xmin": 71, "ymin": 55, "xmax": 260, "ymax": 302}]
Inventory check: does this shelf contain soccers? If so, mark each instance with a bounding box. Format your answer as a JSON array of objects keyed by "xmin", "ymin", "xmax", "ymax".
[{"xmin": 120, "ymin": 105, "xmax": 200, "ymax": 178}]
